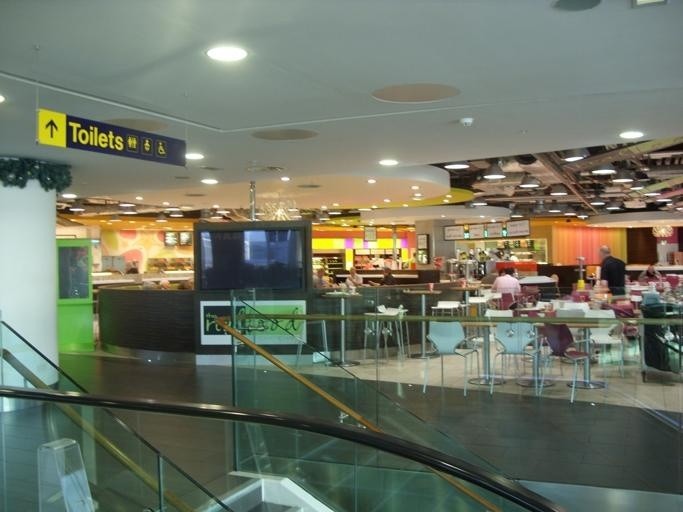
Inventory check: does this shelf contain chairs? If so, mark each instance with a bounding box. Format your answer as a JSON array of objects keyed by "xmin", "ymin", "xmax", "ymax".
[{"xmin": 359, "ymin": 279, "xmax": 682, "ymax": 404}]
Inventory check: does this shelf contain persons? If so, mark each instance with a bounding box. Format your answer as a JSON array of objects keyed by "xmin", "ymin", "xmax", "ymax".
[
  {"xmin": 458, "ymin": 249, "xmax": 521, "ymax": 309},
  {"xmin": 597, "ymin": 246, "xmax": 625, "ymax": 296},
  {"xmin": 314, "ymin": 258, "xmax": 362, "ymax": 288},
  {"xmin": 638, "ymin": 265, "xmax": 661, "ymax": 282},
  {"xmin": 368, "ymin": 267, "xmax": 394, "ymax": 286},
  {"xmin": 126, "ymin": 261, "xmax": 138, "ymax": 274}
]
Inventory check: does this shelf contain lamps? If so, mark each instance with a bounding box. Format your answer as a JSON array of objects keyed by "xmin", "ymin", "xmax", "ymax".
[
  {"xmin": 442, "ymin": 147, "xmax": 682, "ymax": 217},
  {"xmin": 67, "ymin": 200, "xmax": 184, "ymax": 218}
]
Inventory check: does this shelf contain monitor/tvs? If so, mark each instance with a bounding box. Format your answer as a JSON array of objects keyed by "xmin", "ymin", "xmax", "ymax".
[{"xmin": 192, "ymin": 220, "xmax": 312, "ymax": 301}]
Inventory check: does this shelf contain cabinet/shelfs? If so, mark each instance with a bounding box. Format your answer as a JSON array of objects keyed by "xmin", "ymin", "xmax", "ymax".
[
  {"xmin": 352, "ymin": 249, "xmax": 401, "ymax": 269},
  {"xmin": 310, "ymin": 249, "xmax": 346, "ymax": 275}
]
